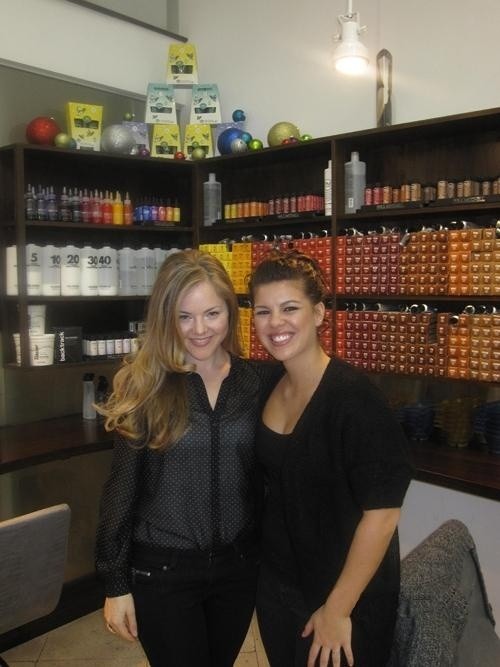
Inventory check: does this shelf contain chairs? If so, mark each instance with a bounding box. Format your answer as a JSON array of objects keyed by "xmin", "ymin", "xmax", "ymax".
[
  {"xmin": 0, "ymin": 503, "xmax": 74, "ymax": 635},
  {"xmin": 390, "ymin": 517, "xmax": 500, "ymax": 666}
]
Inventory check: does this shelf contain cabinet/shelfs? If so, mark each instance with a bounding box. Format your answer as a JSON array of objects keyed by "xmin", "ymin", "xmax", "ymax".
[
  {"xmin": 199, "ymin": 108, "xmax": 500, "ymax": 504},
  {"xmin": 2, "ymin": 143, "xmax": 199, "ymax": 472}
]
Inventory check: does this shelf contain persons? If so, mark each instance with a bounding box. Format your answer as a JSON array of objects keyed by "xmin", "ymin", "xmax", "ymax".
[
  {"xmin": 94, "ymin": 249, "xmax": 275, "ymax": 667},
  {"xmin": 246, "ymin": 250, "xmax": 413, "ymax": 667}
]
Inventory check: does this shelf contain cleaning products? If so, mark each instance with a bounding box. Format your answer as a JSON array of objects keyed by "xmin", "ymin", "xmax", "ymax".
[
  {"xmin": 6, "ymin": 242, "xmax": 182, "ymax": 299},
  {"xmin": 202, "ymin": 172, "xmax": 224, "ymax": 227},
  {"xmin": 344, "ymin": 151, "xmax": 365, "ymax": 214},
  {"xmin": 322, "ymin": 159, "xmax": 332, "ymax": 216}
]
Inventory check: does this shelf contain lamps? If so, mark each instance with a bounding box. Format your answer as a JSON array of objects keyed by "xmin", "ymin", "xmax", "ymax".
[{"xmin": 328, "ymin": 0, "xmax": 377, "ymax": 80}]
